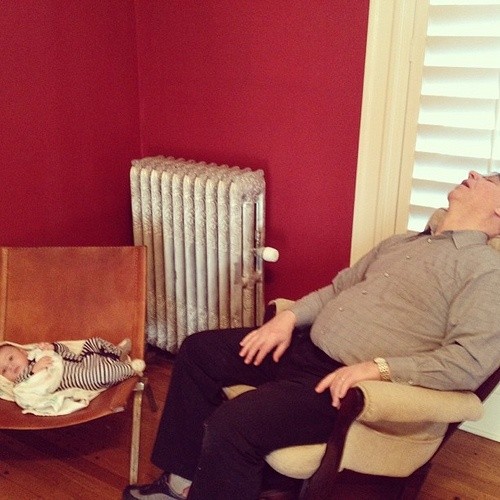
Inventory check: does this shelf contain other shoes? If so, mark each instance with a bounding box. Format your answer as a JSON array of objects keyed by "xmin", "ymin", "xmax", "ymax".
[
  {"xmin": 117, "ymin": 337, "xmax": 147, "ymax": 377},
  {"xmin": 122, "ymin": 471, "xmax": 189, "ymax": 500}
]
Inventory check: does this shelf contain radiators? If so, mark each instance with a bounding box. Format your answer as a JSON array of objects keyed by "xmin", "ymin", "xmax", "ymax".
[{"xmin": 128, "ymin": 154, "xmax": 280, "ymax": 355}]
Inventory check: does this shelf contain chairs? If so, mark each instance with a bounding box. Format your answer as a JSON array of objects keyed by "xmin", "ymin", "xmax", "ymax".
[
  {"xmin": 222, "ymin": 208, "xmax": 500, "ymax": 500},
  {"xmin": 0, "ymin": 246, "xmax": 158, "ymax": 487}
]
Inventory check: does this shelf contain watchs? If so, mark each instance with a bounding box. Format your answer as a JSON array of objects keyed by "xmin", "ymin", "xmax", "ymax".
[{"xmin": 373, "ymin": 357, "xmax": 391, "ymax": 382}]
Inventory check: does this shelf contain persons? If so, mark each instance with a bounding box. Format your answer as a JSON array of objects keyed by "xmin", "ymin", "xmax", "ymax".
[
  {"xmin": 122, "ymin": 168, "xmax": 500, "ymax": 500},
  {"xmin": 0, "ymin": 337, "xmax": 146, "ymax": 399}
]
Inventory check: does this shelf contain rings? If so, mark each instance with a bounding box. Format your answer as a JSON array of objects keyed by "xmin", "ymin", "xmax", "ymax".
[{"xmin": 338, "ymin": 377, "xmax": 346, "ymax": 382}]
